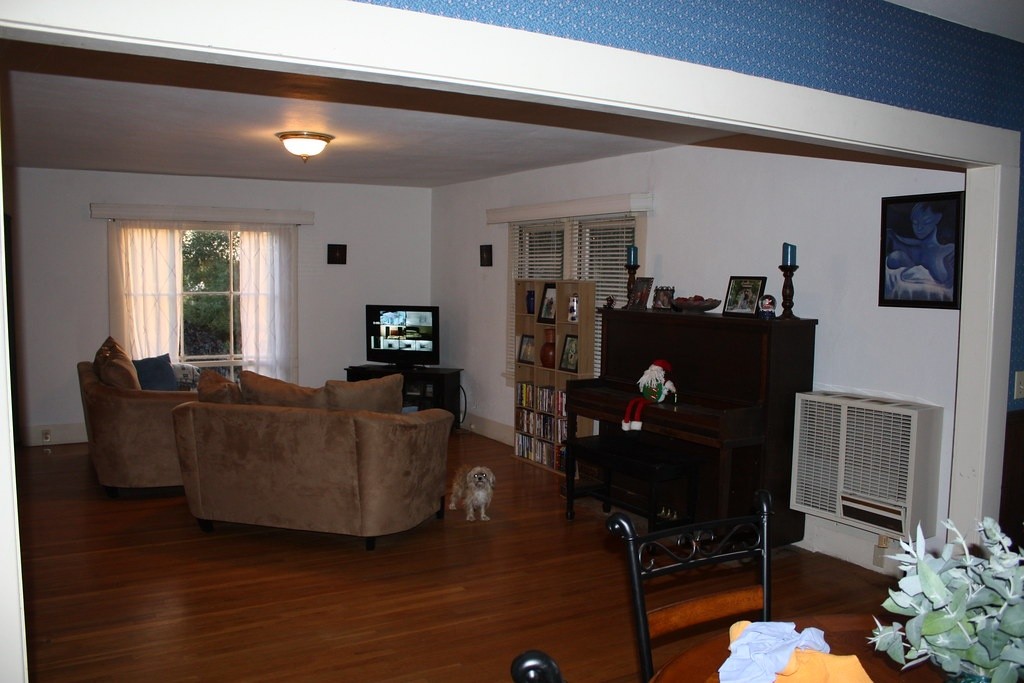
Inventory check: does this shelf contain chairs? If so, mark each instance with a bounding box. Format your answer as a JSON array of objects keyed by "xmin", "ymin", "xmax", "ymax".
[{"xmin": 606, "ymin": 490, "xmax": 775, "ymax": 683}]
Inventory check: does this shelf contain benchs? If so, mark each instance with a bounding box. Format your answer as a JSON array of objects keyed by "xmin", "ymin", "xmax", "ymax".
[{"xmin": 562, "ymin": 434, "xmax": 706, "ymax": 555}]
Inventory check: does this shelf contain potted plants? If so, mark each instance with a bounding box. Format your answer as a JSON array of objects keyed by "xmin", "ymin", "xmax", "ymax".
[{"xmin": 865, "ymin": 516, "xmax": 1024, "ymax": 683}]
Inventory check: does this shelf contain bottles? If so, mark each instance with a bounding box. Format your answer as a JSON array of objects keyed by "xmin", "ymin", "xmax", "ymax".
[
  {"xmin": 526, "ymin": 290, "xmax": 534, "ymax": 314},
  {"xmin": 540, "ymin": 329, "xmax": 555, "ymax": 368}
]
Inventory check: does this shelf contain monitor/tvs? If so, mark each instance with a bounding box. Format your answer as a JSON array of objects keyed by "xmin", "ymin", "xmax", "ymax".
[{"xmin": 365, "ymin": 305, "xmax": 440, "ymax": 371}]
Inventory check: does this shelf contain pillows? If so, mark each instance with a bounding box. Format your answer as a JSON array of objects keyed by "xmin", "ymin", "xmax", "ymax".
[
  {"xmin": 132, "ymin": 353, "xmax": 179, "ymax": 391},
  {"xmin": 326, "ymin": 373, "xmax": 404, "ymax": 413},
  {"xmin": 93, "ymin": 336, "xmax": 142, "ymax": 390},
  {"xmin": 239, "ymin": 370, "xmax": 326, "ymax": 409},
  {"xmin": 197, "ymin": 369, "xmax": 243, "ymax": 404}
]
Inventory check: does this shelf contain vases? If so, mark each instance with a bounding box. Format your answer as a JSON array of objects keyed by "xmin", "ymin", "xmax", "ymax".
[
  {"xmin": 526, "ymin": 290, "xmax": 535, "ymax": 314},
  {"xmin": 540, "ymin": 329, "xmax": 555, "ymax": 369}
]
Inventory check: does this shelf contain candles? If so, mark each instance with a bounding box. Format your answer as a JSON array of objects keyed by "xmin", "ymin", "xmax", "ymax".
[
  {"xmin": 782, "ymin": 242, "xmax": 796, "ymax": 265},
  {"xmin": 627, "ymin": 245, "xmax": 637, "ymax": 265}
]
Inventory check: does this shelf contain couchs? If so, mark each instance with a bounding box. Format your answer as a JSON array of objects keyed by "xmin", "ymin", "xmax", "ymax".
[
  {"xmin": 76, "ymin": 361, "xmax": 199, "ymax": 498},
  {"xmin": 170, "ymin": 401, "xmax": 454, "ymax": 551}
]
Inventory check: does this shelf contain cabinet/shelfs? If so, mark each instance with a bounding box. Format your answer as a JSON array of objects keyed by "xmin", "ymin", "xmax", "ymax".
[
  {"xmin": 515, "ymin": 278, "xmax": 596, "ymax": 480},
  {"xmin": 344, "ymin": 364, "xmax": 464, "ymax": 434}
]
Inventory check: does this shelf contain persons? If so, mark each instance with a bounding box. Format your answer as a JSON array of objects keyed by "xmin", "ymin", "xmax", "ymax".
[
  {"xmin": 568, "ymin": 343, "xmax": 576, "ymax": 363},
  {"xmin": 656, "ymin": 290, "xmax": 671, "ymax": 308},
  {"xmin": 622, "ymin": 359, "xmax": 678, "ymax": 431},
  {"xmin": 543, "ymin": 297, "xmax": 554, "ymax": 318}
]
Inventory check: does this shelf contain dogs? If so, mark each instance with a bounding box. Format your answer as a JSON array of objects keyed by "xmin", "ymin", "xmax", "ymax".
[{"xmin": 448, "ymin": 462, "xmax": 498, "ymax": 521}]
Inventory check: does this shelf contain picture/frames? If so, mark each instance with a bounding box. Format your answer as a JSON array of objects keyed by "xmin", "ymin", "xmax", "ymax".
[
  {"xmin": 627, "ymin": 277, "xmax": 654, "ymax": 310},
  {"xmin": 878, "ymin": 191, "xmax": 965, "ymax": 309},
  {"xmin": 722, "ymin": 276, "xmax": 767, "ymax": 317},
  {"xmin": 518, "ymin": 334, "xmax": 535, "ymax": 365},
  {"xmin": 538, "ymin": 283, "xmax": 556, "ymax": 324},
  {"xmin": 652, "ymin": 286, "xmax": 675, "ymax": 311},
  {"xmin": 559, "ymin": 334, "xmax": 578, "ymax": 373}
]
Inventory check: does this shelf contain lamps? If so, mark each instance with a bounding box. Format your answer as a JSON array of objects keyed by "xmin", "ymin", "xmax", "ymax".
[{"xmin": 278, "ymin": 132, "xmax": 333, "ymax": 164}]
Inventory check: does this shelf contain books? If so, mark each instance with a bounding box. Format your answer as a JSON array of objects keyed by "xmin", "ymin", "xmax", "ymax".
[{"xmin": 515, "ymin": 382, "xmax": 567, "ymax": 473}]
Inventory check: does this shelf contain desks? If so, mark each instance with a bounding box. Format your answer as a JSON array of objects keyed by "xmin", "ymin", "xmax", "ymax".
[{"xmin": 648, "ymin": 613, "xmax": 957, "ymax": 683}]
